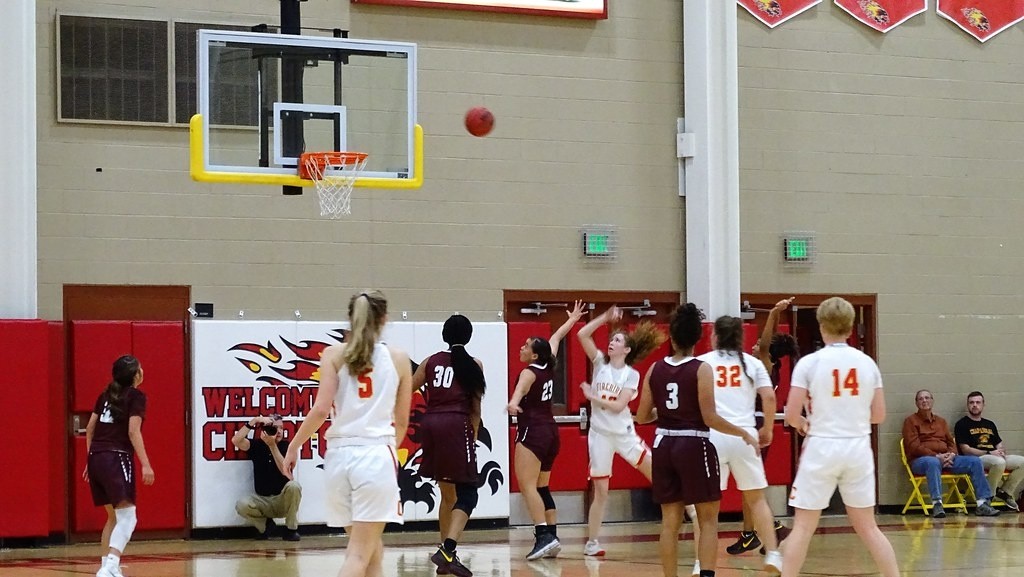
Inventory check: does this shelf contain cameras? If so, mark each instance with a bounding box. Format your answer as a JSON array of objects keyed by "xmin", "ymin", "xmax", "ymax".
[{"xmin": 261, "ymin": 423, "xmax": 277, "ymax": 436}]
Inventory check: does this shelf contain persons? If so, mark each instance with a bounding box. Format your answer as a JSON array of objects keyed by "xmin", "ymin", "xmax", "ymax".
[
  {"xmin": 411, "ymin": 315, "xmax": 486, "ymax": 577},
  {"xmin": 577, "ymin": 305, "xmax": 696, "ymax": 556},
  {"xmin": 232, "ymin": 413, "xmax": 302, "ymax": 540},
  {"xmin": 691, "ymin": 314, "xmax": 783, "ymax": 577},
  {"xmin": 780, "ymin": 297, "xmax": 902, "ymax": 577},
  {"xmin": 504, "ymin": 298, "xmax": 589, "ymax": 560},
  {"xmin": 954, "ymin": 391, "xmax": 1023, "ymax": 509},
  {"xmin": 282, "ymin": 289, "xmax": 413, "ymax": 576},
  {"xmin": 902, "ymin": 390, "xmax": 1001, "ymax": 518},
  {"xmin": 637, "ymin": 302, "xmax": 760, "ymax": 577},
  {"xmin": 725, "ymin": 296, "xmax": 793, "ymax": 562},
  {"xmin": 82, "ymin": 355, "xmax": 155, "ymax": 577}
]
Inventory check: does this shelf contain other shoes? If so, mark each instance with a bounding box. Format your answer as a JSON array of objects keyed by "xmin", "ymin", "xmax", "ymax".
[{"xmin": 96, "ymin": 556, "xmax": 123, "ymax": 577}]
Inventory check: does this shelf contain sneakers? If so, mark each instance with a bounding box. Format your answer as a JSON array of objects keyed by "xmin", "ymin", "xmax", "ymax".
[
  {"xmin": 760, "ymin": 520, "xmax": 788, "ymax": 554},
  {"xmin": 763, "ymin": 551, "xmax": 782, "ymax": 574},
  {"xmin": 256, "ymin": 519, "xmax": 277, "ymax": 541},
  {"xmin": 932, "ymin": 502, "xmax": 946, "ymax": 517},
  {"xmin": 437, "ymin": 564, "xmax": 452, "ymax": 575},
  {"xmin": 727, "ymin": 530, "xmax": 761, "ymax": 555},
  {"xmin": 994, "ymin": 487, "xmax": 1019, "ymax": 510},
  {"xmin": 283, "ymin": 529, "xmax": 301, "ymax": 541},
  {"xmin": 542, "ymin": 535, "xmax": 562, "ymax": 559},
  {"xmin": 692, "ymin": 560, "xmax": 700, "ymax": 576},
  {"xmin": 524, "ymin": 531, "xmax": 559, "ymax": 560},
  {"xmin": 431, "ymin": 542, "xmax": 474, "ymax": 577},
  {"xmin": 975, "ymin": 503, "xmax": 1000, "ymax": 516},
  {"xmin": 584, "ymin": 541, "xmax": 605, "ymax": 557}
]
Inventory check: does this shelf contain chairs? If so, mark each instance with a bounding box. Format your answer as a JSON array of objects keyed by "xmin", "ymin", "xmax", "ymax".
[{"xmin": 900, "ymin": 438, "xmax": 1012, "ymax": 516}]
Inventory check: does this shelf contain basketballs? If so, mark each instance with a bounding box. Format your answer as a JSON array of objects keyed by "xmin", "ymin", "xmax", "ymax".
[{"xmin": 465, "ymin": 107, "xmax": 494, "ymax": 136}]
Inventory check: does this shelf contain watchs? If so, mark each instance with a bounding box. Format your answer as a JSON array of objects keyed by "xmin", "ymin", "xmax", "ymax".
[{"xmin": 986, "ymin": 451, "xmax": 991, "ymax": 455}]
